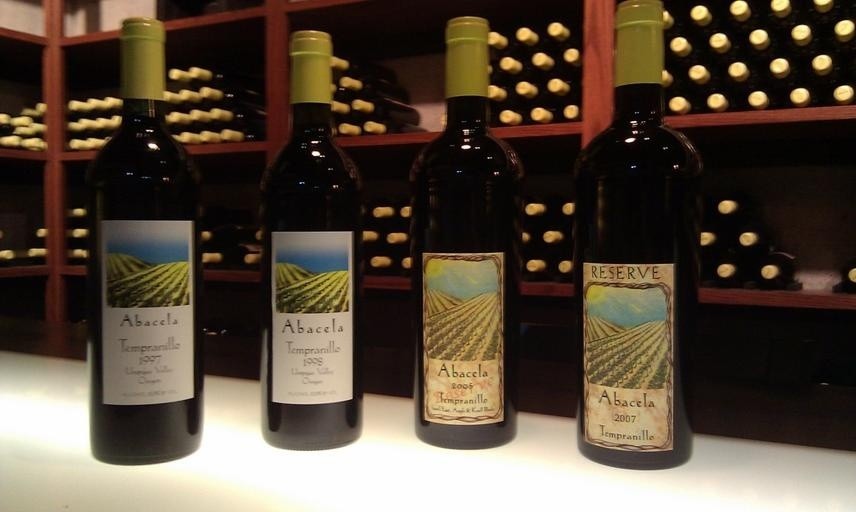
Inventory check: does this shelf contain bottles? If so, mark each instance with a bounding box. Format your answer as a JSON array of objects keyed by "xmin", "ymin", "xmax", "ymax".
[
  {"xmin": 333, "ymin": 51, "xmax": 426, "ymax": 133},
  {"xmin": 411, "ymin": 16, "xmax": 524, "ymax": 450},
  {"xmin": 170, "ymin": 64, "xmax": 266, "ymax": 142},
  {"xmin": 86, "ymin": 17, "xmax": 207, "ymax": 465},
  {"xmin": 574, "ymin": 0, "xmax": 704, "ymax": 468},
  {"xmin": 262, "ymin": 27, "xmax": 365, "ymax": 451},
  {"xmin": 201, "ymin": 223, "xmax": 261, "ymax": 271}
]
